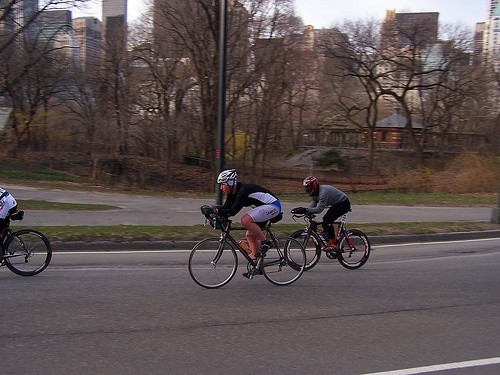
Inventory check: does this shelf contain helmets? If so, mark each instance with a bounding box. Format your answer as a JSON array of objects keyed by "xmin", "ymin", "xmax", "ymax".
[
  {"xmin": 303, "ymin": 175, "xmax": 319, "ymax": 197},
  {"xmin": 217, "ymin": 169, "xmax": 238, "ymax": 189}
]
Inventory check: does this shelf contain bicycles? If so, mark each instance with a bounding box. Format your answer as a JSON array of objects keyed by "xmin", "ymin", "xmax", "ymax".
[
  {"xmin": 188, "ymin": 204, "xmax": 307, "ymax": 289},
  {"xmin": 283, "ymin": 207, "xmax": 371, "ymax": 272},
  {"xmin": 0, "ymin": 207, "xmax": 53, "ymax": 276}
]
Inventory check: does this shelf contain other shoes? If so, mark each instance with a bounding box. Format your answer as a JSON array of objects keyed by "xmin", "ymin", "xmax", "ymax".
[{"xmin": 323, "ymin": 239, "xmax": 337, "ymax": 252}]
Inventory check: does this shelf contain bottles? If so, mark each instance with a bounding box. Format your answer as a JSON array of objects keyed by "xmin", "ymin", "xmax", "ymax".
[
  {"xmin": 239, "ymin": 241, "xmax": 252, "ymax": 255},
  {"xmin": 256, "ymin": 239, "xmax": 261, "ymax": 249}
]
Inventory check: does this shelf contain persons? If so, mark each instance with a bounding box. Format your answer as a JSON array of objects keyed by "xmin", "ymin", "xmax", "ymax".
[
  {"xmin": 0, "ymin": 186, "xmax": 18, "ymax": 267},
  {"xmin": 201, "ymin": 169, "xmax": 281, "ymax": 276},
  {"xmin": 291, "ymin": 176, "xmax": 351, "ymax": 253}
]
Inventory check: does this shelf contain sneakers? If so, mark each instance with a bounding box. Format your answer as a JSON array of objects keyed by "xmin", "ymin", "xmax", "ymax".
[
  {"xmin": 254, "ymin": 240, "xmax": 273, "ymax": 257},
  {"xmin": 242, "ymin": 267, "xmax": 263, "ymax": 278}
]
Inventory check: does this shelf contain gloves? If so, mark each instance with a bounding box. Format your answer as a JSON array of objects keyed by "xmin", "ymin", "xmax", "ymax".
[
  {"xmin": 201, "ymin": 205, "xmax": 217, "ymax": 217},
  {"xmin": 291, "ymin": 207, "xmax": 307, "ymax": 214}
]
